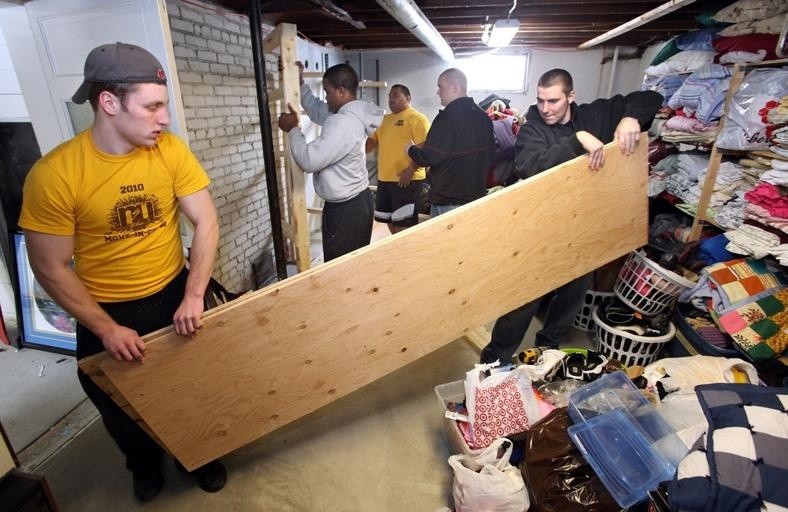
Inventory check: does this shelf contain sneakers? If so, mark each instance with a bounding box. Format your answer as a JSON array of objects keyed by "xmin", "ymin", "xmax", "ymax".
[
  {"xmin": 133, "ymin": 469, "xmax": 165, "ymax": 500},
  {"xmin": 175, "ymin": 457, "xmax": 228, "ymax": 492}
]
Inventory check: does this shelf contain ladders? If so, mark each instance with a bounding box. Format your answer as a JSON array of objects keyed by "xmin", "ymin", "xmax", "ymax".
[{"xmin": 262, "ymin": 22, "xmax": 311, "ymax": 275}]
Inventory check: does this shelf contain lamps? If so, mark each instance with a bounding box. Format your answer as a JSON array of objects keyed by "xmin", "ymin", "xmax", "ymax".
[{"xmin": 486, "ymin": 0, "xmax": 522, "ymax": 49}]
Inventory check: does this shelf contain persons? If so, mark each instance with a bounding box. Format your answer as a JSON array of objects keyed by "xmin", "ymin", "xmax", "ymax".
[
  {"xmin": 404, "ymin": 68, "xmax": 495, "ymax": 219},
  {"xmin": 478, "ymin": 68, "xmax": 665, "ymax": 368},
  {"xmin": 17, "ymin": 43, "xmax": 227, "ymax": 503},
  {"xmin": 277, "ymin": 55, "xmax": 385, "ymax": 262},
  {"xmin": 364, "ymin": 84, "xmax": 430, "ymax": 233}
]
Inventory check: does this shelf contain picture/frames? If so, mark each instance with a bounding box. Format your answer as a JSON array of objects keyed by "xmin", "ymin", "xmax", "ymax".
[{"xmin": 9, "ymin": 228, "xmax": 77, "ymax": 358}]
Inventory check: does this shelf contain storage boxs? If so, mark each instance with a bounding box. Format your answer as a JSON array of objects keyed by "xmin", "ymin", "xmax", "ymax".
[
  {"xmin": 565, "ymin": 369, "xmax": 691, "ymax": 509},
  {"xmin": 432, "ymin": 378, "xmax": 487, "ymax": 457}
]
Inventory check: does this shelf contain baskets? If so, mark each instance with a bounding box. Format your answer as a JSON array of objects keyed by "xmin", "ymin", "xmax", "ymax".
[
  {"xmin": 614, "ymin": 249, "xmax": 696, "ymax": 318},
  {"xmin": 572, "ymin": 288, "xmax": 675, "ymax": 368}
]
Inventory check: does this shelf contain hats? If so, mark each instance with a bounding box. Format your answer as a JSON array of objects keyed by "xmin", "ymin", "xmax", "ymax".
[{"xmin": 71, "ymin": 42, "xmax": 166, "ymax": 104}]
